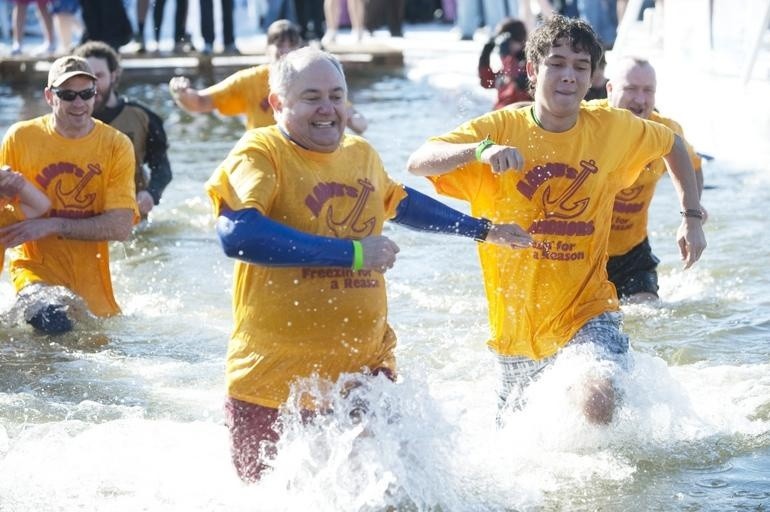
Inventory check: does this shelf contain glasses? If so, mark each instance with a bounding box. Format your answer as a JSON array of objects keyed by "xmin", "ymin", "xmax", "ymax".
[{"xmin": 51, "ymin": 88, "xmax": 97, "ymax": 102}]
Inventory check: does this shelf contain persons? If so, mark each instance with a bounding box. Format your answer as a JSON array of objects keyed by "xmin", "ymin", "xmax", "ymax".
[
  {"xmin": 406, "ymin": 11, "xmax": 710, "ymax": 429},
  {"xmin": 168, "ymin": 19, "xmax": 369, "ymax": 139},
  {"xmin": 0, "ymin": 1, "xmax": 657, "ymax": 58},
  {"xmin": 478, "ymin": 17, "xmax": 537, "ymax": 112},
  {"xmin": 2, "ymin": 56, "xmax": 144, "ymax": 320},
  {"xmin": 584, "ymin": 38, "xmax": 662, "ymax": 116},
  {"xmin": 203, "ymin": 45, "xmax": 534, "ymax": 484},
  {"xmin": 73, "ymin": 40, "xmax": 174, "ymax": 233},
  {"xmin": 503, "ymin": 55, "xmax": 709, "ymax": 304},
  {"xmin": 2, "ymin": 169, "xmax": 54, "ymax": 276}
]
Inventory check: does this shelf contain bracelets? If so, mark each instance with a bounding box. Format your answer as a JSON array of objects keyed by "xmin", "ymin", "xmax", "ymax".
[
  {"xmin": 680, "ymin": 209, "xmax": 703, "ymax": 221},
  {"xmin": 474, "ymin": 216, "xmax": 493, "ymax": 245},
  {"xmin": 352, "ymin": 239, "xmax": 365, "ymax": 272},
  {"xmin": 476, "ymin": 131, "xmax": 497, "ymax": 162}
]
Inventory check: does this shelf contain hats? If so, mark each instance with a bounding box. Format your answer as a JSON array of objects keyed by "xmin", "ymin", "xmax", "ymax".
[{"xmin": 47, "ymin": 55, "xmax": 98, "ymax": 87}]
